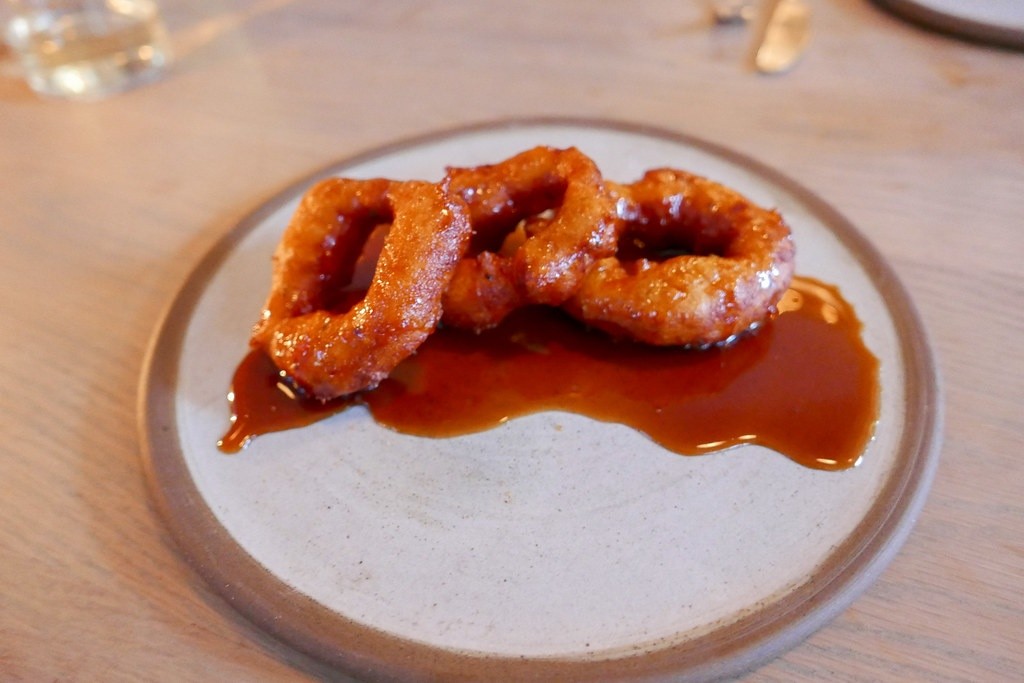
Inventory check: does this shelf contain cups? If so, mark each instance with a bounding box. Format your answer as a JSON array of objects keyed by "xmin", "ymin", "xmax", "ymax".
[{"xmin": 0, "ymin": 0, "xmax": 170, "ymax": 102}]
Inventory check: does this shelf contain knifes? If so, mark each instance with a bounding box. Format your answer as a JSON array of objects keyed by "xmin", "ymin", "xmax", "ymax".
[{"xmin": 754, "ymin": 0, "xmax": 814, "ymax": 74}]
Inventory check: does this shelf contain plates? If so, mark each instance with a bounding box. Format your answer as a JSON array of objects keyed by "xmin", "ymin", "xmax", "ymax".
[
  {"xmin": 139, "ymin": 114, "xmax": 944, "ymax": 683},
  {"xmin": 870, "ymin": 0, "xmax": 1024, "ymax": 54}
]
